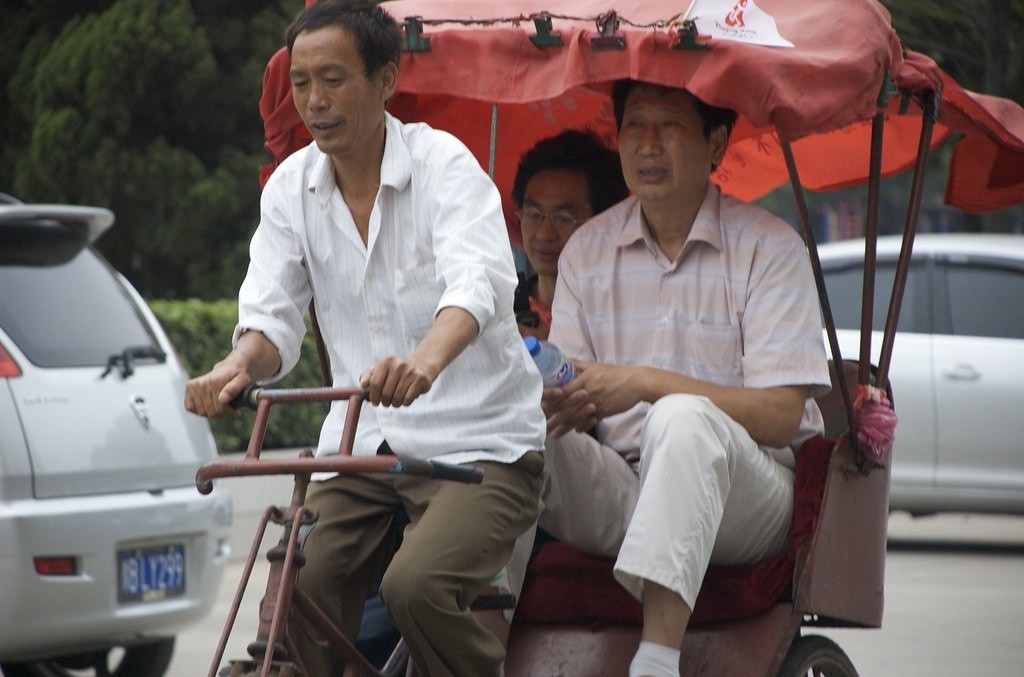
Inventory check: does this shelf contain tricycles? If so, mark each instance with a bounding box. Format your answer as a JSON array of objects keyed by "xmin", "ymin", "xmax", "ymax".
[{"xmin": 195, "ymin": 0, "xmax": 1024, "ymax": 676}]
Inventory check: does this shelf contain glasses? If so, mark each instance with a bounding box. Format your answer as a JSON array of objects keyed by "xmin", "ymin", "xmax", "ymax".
[{"xmin": 515, "ymin": 208, "xmax": 584, "ymax": 228}]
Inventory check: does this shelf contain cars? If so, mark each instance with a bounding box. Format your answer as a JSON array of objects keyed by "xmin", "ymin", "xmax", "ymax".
[
  {"xmin": 804, "ymin": 232, "xmax": 1023, "ymax": 522},
  {"xmin": 0, "ymin": 190, "xmax": 236, "ymax": 677}
]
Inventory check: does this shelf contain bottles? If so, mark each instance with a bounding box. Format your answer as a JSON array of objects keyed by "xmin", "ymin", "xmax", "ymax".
[{"xmin": 521, "ymin": 336, "xmax": 579, "ymax": 389}]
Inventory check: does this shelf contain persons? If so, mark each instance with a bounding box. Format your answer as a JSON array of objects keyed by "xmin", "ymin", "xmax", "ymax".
[
  {"xmin": 405, "ymin": 79, "xmax": 831, "ymax": 677},
  {"xmin": 185, "ymin": 0, "xmax": 548, "ymax": 677},
  {"xmin": 359, "ymin": 128, "xmax": 629, "ymax": 648}
]
